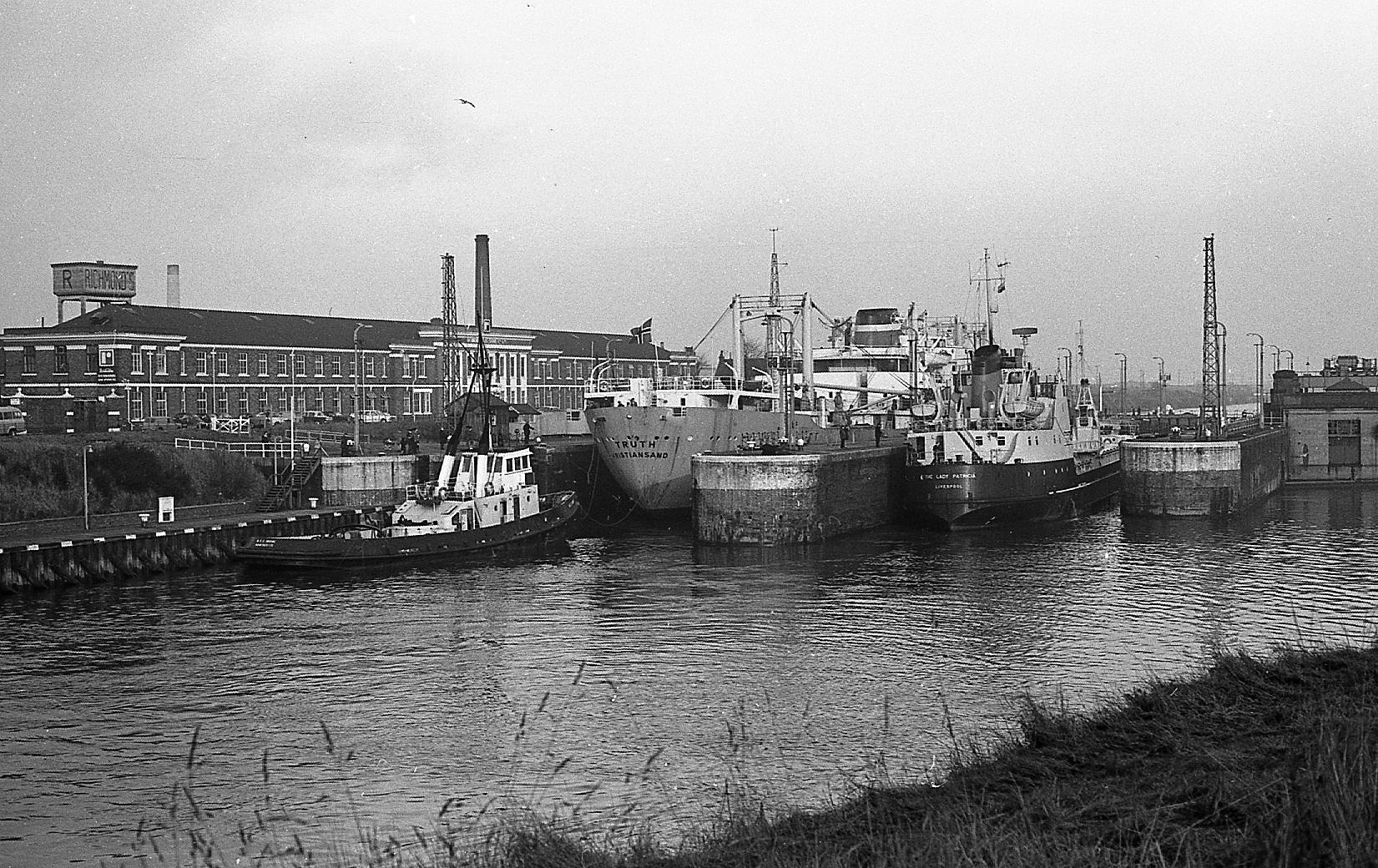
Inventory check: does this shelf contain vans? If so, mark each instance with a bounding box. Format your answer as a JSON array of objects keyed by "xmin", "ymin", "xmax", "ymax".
[{"xmin": 0, "ymin": 407, "xmax": 27, "ymax": 437}]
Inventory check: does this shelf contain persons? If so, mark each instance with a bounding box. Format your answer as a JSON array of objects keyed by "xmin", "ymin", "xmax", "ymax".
[
  {"xmin": 465, "ymin": 426, "xmax": 474, "ymax": 450},
  {"xmin": 874, "ymin": 423, "xmax": 883, "ymax": 448},
  {"xmin": 340, "ymin": 433, "xmax": 349, "ymax": 457},
  {"xmin": 400, "ymin": 428, "xmax": 420, "ymax": 455},
  {"xmin": 439, "ymin": 427, "xmax": 449, "ymax": 450},
  {"xmin": 261, "ymin": 431, "xmax": 269, "ymax": 456},
  {"xmin": 585, "ymin": 396, "xmax": 638, "ymax": 410},
  {"xmin": 839, "ymin": 424, "xmax": 849, "ymax": 448},
  {"xmin": 523, "ymin": 420, "xmax": 535, "ymax": 444}
]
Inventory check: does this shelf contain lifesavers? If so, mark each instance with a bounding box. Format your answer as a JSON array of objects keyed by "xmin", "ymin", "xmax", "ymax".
[
  {"xmin": 600, "ymin": 380, "xmax": 609, "ymax": 391},
  {"xmin": 701, "ymin": 378, "xmax": 710, "ymax": 389},
  {"xmin": 484, "ymin": 482, "xmax": 493, "ymax": 495},
  {"xmin": 438, "ymin": 487, "xmax": 447, "ymax": 500}
]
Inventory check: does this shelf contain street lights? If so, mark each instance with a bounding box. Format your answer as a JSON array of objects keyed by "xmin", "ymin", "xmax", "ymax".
[
  {"xmin": 1152, "ymin": 356, "xmax": 1165, "ymax": 410},
  {"xmin": 1114, "ymin": 352, "xmax": 1127, "ymax": 413},
  {"xmin": 1057, "ymin": 347, "xmax": 1072, "ymax": 386},
  {"xmin": 1246, "ymin": 333, "xmax": 1264, "ymax": 402},
  {"xmin": 1265, "ymin": 344, "xmax": 1279, "ymax": 370},
  {"xmin": 1281, "ymin": 350, "xmax": 1294, "ymax": 370}
]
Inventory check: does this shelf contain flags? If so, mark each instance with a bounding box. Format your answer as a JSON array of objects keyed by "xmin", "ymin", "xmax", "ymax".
[
  {"xmin": 998, "ymin": 281, "xmax": 1006, "ymax": 293},
  {"xmin": 629, "ymin": 318, "xmax": 655, "ymax": 344}
]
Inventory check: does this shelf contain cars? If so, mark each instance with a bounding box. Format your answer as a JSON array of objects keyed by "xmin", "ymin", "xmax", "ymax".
[{"xmin": 140, "ymin": 409, "xmax": 398, "ymax": 433}]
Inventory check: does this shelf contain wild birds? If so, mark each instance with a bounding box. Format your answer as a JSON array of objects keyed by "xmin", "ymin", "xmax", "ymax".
[{"xmin": 456, "ymin": 98, "xmax": 476, "ymax": 108}]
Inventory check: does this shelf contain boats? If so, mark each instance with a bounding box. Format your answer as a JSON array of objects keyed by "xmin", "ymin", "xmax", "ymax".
[
  {"xmin": 583, "ymin": 228, "xmax": 916, "ymax": 520},
  {"xmin": 235, "ymin": 304, "xmax": 580, "ymax": 567},
  {"xmin": 903, "ymin": 248, "xmax": 1138, "ymax": 532}
]
